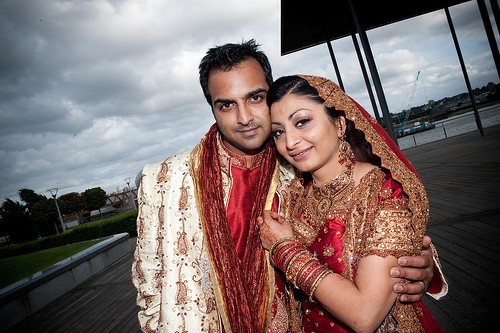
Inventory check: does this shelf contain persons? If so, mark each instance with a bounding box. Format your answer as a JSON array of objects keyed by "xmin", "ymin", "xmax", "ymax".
[
  {"xmin": 130, "ymin": 43, "xmax": 449, "ymax": 333},
  {"xmin": 256, "ymin": 75, "xmax": 443, "ymax": 333}
]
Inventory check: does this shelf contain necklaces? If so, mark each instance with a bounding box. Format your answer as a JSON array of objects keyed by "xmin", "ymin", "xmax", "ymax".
[{"xmin": 311, "ymin": 158, "xmax": 357, "ymax": 219}]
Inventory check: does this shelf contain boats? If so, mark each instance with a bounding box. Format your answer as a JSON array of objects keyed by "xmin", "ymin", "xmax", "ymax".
[{"xmin": 394, "ymin": 119, "xmax": 436, "ymax": 138}]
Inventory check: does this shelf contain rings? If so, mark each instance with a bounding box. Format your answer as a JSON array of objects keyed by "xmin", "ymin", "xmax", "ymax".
[{"xmin": 419, "ymin": 280, "xmax": 429, "ymax": 294}]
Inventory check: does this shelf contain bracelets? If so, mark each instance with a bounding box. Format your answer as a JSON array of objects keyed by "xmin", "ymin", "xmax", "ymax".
[{"xmin": 269, "ymin": 238, "xmax": 333, "ymax": 303}]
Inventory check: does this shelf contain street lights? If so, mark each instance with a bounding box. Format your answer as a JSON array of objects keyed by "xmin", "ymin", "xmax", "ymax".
[
  {"xmin": 123, "ymin": 177, "xmax": 138, "ymax": 211},
  {"xmin": 47, "ymin": 187, "xmax": 67, "ymax": 232}
]
